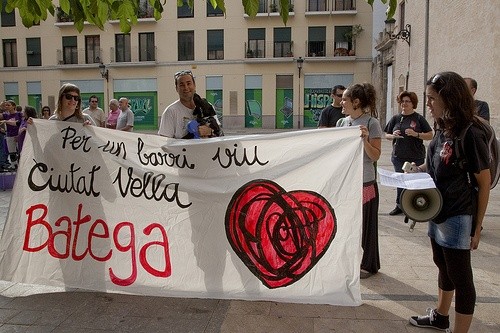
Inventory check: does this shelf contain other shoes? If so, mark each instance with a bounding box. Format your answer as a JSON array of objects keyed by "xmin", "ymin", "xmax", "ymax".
[
  {"xmin": 389, "ymin": 206, "xmax": 404, "ymax": 215},
  {"xmin": 360, "ymin": 270, "xmax": 372, "ymax": 279},
  {"xmin": 0, "ymin": 161, "xmax": 11, "ymax": 173},
  {"xmin": 4, "ymin": 164, "xmax": 17, "ymax": 172}
]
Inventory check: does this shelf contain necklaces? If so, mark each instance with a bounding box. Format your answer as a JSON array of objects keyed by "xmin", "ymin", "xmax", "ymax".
[{"xmin": 348, "ymin": 110, "xmax": 365, "ymax": 126}]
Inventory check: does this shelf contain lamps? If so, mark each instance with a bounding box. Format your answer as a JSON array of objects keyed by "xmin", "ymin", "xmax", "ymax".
[
  {"xmin": 99, "ymin": 63, "xmax": 108, "ymax": 82},
  {"xmin": 297, "ymin": 57, "xmax": 303, "ymax": 79},
  {"xmin": 384, "ymin": 18, "xmax": 411, "ymax": 47}
]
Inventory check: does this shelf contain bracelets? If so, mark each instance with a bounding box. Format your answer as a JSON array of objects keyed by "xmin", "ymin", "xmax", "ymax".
[{"xmin": 416, "ymin": 132, "xmax": 419, "ymax": 138}]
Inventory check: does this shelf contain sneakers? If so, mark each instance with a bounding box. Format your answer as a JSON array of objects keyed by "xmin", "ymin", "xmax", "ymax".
[{"xmin": 409, "ymin": 307, "xmax": 450, "ymax": 330}]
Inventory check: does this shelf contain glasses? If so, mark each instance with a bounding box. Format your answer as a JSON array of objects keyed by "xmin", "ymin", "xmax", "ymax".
[
  {"xmin": 432, "ymin": 73, "xmax": 447, "ymax": 86},
  {"xmin": 400, "ymin": 100, "xmax": 412, "ymax": 103},
  {"xmin": 174, "ymin": 70, "xmax": 193, "ymax": 81},
  {"xmin": 43, "ymin": 109, "xmax": 49, "ymax": 111},
  {"xmin": 90, "ymin": 100, "xmax": 98, "ymax": 103},
  {"xmin": 63, "ymin": 93, "xmax": 79, "ymax": 101},
  {"xmin": 334, "ymin": 93, "xmax": 343, "ymax": 97}
]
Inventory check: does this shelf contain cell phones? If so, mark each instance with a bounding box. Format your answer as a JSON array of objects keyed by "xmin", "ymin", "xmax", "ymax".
[{"xmin": 402, "ymin": 162, "xmax": 417, "ymax": 174}]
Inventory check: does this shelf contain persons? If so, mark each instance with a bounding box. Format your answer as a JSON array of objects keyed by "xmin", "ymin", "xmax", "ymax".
[
  {"xmin": 157, "ymin": 70, "xmax": 224, "ymax": 139},
  {"xmin": 82, "ymin": 95, "xmax": 135, "ymax": 133},
  {"xmin": 26, "ymin": 82, "xmax": 98, "ymax": 128},
  {"xmin": 0, "ymin": 98, "xmax": 53, "ymax": 174},
  {"xmin": 316, "ymin": 72, "xmax": 492, "ymax": 333},
  {"xmin": 333, "ymin": 82, "xmax": 382, "ymax": 279}
]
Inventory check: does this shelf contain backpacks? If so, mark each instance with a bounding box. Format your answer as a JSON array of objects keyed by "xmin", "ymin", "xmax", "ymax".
[{"xmin": 459, "ymin": 114, "xmax": 500, "ymax": 191}]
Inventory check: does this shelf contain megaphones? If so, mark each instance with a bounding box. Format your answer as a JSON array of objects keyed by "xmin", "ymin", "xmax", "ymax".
[{"xmin": 400, "ymin": 189, "xmax": 443, "ymax": 222}]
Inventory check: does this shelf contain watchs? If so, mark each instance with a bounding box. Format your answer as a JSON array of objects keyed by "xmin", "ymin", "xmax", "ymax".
[
  {"xmin": 210, "ymin": 128, "xmax": 215, "ymax": 136},
  {"xmin": 108, "ymin": 124, "xmax": 112, "ymax": 127}
]
